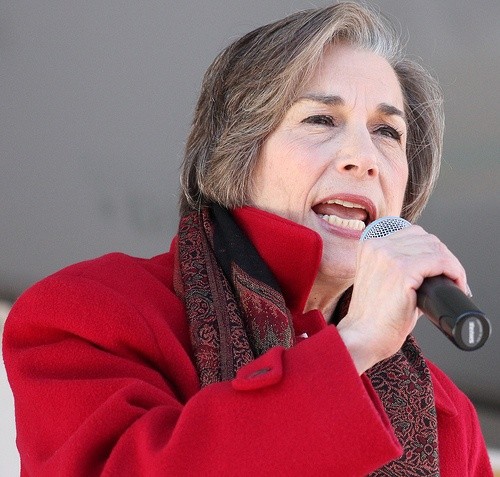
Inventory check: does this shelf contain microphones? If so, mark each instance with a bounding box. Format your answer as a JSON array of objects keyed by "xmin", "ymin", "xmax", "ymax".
[{"xmin": 360, "ymin": 218, "xmax": 493, "ymax": 350}]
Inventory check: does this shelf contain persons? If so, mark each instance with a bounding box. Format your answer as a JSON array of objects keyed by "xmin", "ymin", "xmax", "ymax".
[{"xmin": 1, "ymin": 0, "xmax": 495, "ymax": 477}]
